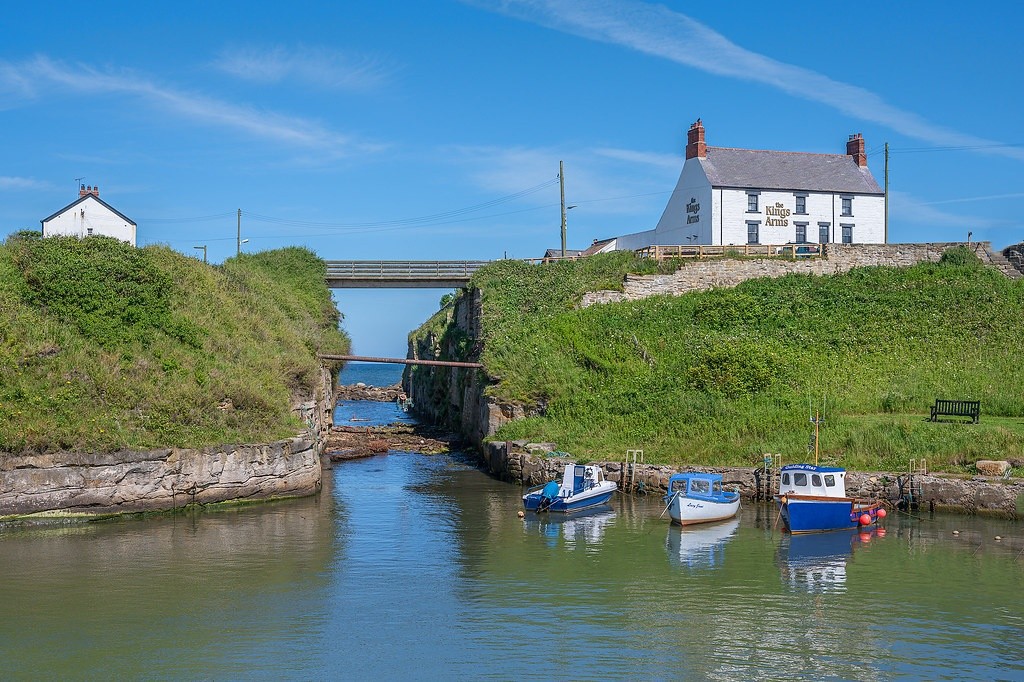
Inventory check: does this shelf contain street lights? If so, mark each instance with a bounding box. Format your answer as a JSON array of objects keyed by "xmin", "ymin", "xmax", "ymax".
[
  {"xmin": 237, "ymin": 239, "xmax": 249, "ymax": 253},
  {"xmin": 561, "ymin": 205, "xmax": 578, "ymax": 257},
  {"xmin": 194, "ymin": 245, "xmax": 206, "ymax": 263}
]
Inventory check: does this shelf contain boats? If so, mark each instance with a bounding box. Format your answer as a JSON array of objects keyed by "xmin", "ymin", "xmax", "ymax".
[
  {"xmin": 664, "ymin": 471, "xmax": 742, "ymax": 526},
  {"xmin": 774, "ymin": 388, "xmax": 880, "ymax": 534},
  {"xmin": 776, "ymin": 523, "xmax": 881, "ymax": 594},
  {"xmin": 523, "ymin": 462, "xmax": 619, "ymax": 514},
  {"xmin": 666, "ymin": 518, "xmax": 745, "ymax": 567}
]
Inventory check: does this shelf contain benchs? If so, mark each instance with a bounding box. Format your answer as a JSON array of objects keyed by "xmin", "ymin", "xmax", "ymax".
[{"xmin": 927, "ymin": 398, "xmax": 980, "ymax": 426}]
